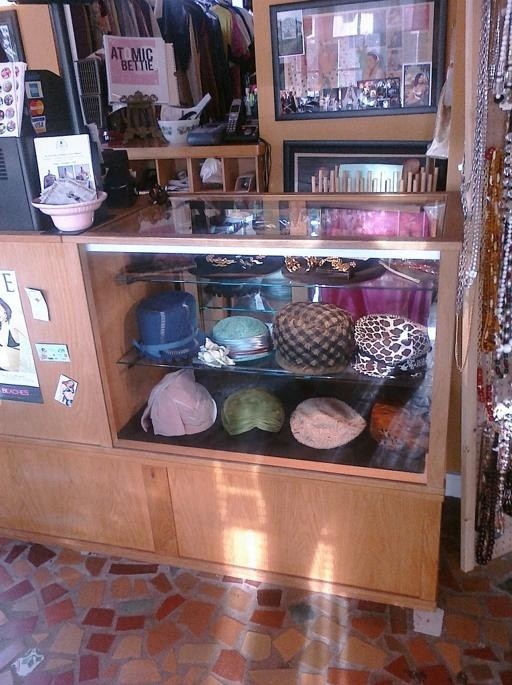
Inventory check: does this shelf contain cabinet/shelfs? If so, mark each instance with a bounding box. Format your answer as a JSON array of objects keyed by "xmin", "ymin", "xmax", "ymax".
[{"xmin": 99, "ymin": 145, "xmax": 266, "ymax": 194}]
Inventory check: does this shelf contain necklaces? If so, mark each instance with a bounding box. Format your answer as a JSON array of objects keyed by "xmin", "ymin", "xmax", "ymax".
[{"xmin": 454, "ymin": 0, "xmax": 512, "ymax": 567}]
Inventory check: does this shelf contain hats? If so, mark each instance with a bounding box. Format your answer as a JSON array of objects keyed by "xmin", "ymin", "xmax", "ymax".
[
  {"xmin": 353, "ymin": 314, "xmax": 431, "ymax": 380},
  {"xmin": 141, "ymin": 369, "xmax": 217, "ymax": 436},
  {"xmin": 272, "ymin": 302, "xmax": 354, "ymax": 374},
  {"xmin": 198, "ymin": 316, "xmax": 274, "ymax": 368},
  {"xmin": 290, "ymin": 398, "xmax": 366, "ymax": 449},
  {"xmin": 132, "ymin": 291, "xmax": 206, "ymax": 364},
  {"xmin": 370, "ymin": 399, "xmax": 430, "ymax": 459},
  {"xmin": 220, "ymin": 388, "xmax": 285, "ymax": 436}
]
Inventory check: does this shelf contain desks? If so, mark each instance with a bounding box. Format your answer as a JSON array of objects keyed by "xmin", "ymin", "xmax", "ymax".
[{"xmin": 1, "ymin": 192, "xmax": 463, "ymax": 613}]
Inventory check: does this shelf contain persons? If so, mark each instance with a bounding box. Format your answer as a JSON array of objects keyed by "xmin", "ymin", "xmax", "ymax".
[
  {"xmin": 62, "ymin": 381, "xmax": 75, "ymax": 407},
  {"xmin": 279, "ymin": 9, "xmax": 430, "ymax": 114},
  {"xmin": 0, "ymin": 297, "xmax": 29, "ymax": 373}
]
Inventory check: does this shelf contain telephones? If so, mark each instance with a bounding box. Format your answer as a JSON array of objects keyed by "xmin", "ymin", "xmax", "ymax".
[{"xmin": 225, "ymin": 98, "xmax": 258, "ymax": 143}]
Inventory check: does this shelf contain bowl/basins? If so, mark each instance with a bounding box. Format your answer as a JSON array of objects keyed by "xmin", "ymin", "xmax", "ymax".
[{"xmin": 157, "ymin": 120, "xmax": 201, "ymax": 145}]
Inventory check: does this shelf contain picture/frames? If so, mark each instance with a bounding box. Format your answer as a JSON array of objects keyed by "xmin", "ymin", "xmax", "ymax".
[
  {"xmin": 269, "ymin": 0, "xmax": 447, "ymax": 121},
  {"xmin": 283, "ymin": 140, "xmax": 448, "ymax": 193}
]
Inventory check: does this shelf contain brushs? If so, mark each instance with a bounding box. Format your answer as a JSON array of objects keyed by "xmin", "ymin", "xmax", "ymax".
[{"xmin": 311, "ymin": 166, "xmax": 439, "ymax": 194}]
